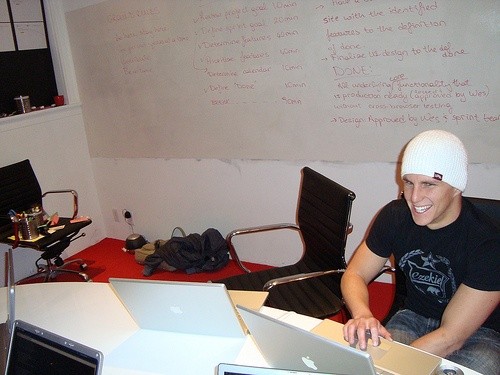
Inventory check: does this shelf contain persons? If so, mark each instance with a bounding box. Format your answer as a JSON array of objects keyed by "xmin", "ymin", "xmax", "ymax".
[{"xmin": 340, "ymin": 130, "xmax": 500, "ymax": 375}]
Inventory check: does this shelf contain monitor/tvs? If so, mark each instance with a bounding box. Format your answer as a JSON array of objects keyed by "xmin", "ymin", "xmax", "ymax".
[
  {"xmin": 5, "ymin": 320, "xmax": 104, "ymax": 375},
  {"xmin": 217, "ymin": 363, "xmax": 320, "ymax": 375}
]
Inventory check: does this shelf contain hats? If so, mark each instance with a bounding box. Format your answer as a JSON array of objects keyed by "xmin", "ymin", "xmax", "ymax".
[{"xmin": 400, "ymin": 130, "xmax": 468, "ymax": 193}]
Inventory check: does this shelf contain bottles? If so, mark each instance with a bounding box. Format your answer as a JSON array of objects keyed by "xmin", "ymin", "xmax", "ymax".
[{"xmin": 14, "ymin": 94, "xmax": 31, "ymax": 114}]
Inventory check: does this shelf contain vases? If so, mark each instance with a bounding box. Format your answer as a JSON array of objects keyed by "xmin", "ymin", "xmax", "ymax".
[{"xmin": 54, "ymin": 95, "xmax": 64, "ymax": 106}]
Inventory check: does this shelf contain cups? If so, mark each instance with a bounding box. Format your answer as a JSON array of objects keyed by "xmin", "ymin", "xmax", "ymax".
[
  {"xmin": 19, "ymin": 217, "xmax": 39, "ymax": 240},
  {"xmin": 54, "ymin": 95, "xmax": 64, "ymax": 106},
  {"xmin": 32, "ymin": 210, "xmax": 44, "ymax": 226}
]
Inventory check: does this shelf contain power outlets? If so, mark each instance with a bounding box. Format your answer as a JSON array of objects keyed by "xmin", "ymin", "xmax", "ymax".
[
  {"xmin": 127, "ymin": 212, "xmax": 135, "ymax": 225},
  {"xmin": 112, "ymin": 208, "xmax": 120, "ymax": 222}
]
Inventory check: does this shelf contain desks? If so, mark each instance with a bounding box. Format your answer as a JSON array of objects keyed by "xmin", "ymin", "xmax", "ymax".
[{"xmin": 0, "ymin": 282, "xmax": 484, "ymax": 375}]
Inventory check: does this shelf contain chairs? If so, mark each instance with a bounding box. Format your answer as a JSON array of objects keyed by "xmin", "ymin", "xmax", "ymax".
[
  {"xmin": 342, "ymin": 190, "xmax": 500, "ymax": 334},
  {"xmin": 210, "ymin": 167, "xmax": 356, "ymax": 326},
  {"xmin": 0, "ymin": 159, "xmax": 92, "ymax": 285}
]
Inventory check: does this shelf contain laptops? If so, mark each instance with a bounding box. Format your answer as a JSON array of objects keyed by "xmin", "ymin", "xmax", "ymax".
[
  {"xmin": 107, "ymin": 277, "xmax": 269, "ymax": 335},
  {"xmin": 237, "ymin": 303, "xmax": 442, "ymax": 374},
  {"xmin": 0, "ymin": 248, "xmax": 14, "ymax": 375}
]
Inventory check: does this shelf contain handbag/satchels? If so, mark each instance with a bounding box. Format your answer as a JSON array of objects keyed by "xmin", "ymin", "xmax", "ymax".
[{"xmin": 143, "ymin": 228, "xmax": 229, "ymax": 277}]
